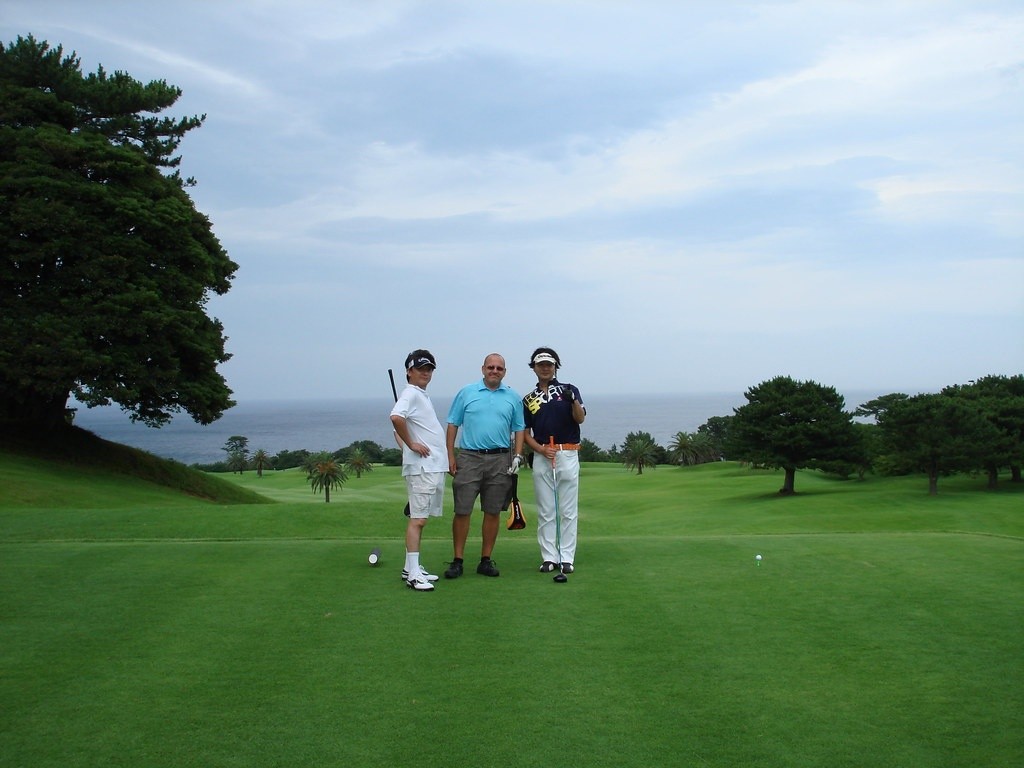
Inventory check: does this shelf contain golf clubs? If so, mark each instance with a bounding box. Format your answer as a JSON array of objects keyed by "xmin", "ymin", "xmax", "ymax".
[
  {"xmin": 387, "ymin": 368, "xmax": 411, "ymax": 519},
  {"xmin": 549, "ymin": 434, "xmax": 566, "ymax": 583}
]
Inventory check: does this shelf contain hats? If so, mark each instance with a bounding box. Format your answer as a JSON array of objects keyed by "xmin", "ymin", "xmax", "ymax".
[
  {"xmin": 532, "ymin": 351, "xmax": 558, "ymax": 364},
  {"xmin": 407, "ymin": 356, "xmax": 436, "ymax": 370}
]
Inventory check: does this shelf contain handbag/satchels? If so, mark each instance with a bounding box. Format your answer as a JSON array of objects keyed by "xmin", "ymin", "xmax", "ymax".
[{"xmin": 507, "ymin": 473, "xmax": 526, "ymax": 530}]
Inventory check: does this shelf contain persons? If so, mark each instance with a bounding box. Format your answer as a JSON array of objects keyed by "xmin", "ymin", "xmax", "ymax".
[
  {"xmin": 521, "ymin": 348, "xmax": 587, "ymax": 574},
  {"xmin": 389, "ymin": 348, "xmax": 450, "ymax": 590},
  {"xmin": 442, "ymin": 353, "xmax": 526, "ymax": 578}
]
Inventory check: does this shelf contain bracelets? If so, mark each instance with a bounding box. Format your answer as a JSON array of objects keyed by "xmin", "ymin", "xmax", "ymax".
[{"xmin": 512, "ymin": 453, "xmax": 525, "ymax": 464}]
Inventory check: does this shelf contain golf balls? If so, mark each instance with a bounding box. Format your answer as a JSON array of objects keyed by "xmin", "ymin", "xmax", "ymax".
[{"xmin": 368, "ymin": 553, "xmax": 378, "ymax": 567}]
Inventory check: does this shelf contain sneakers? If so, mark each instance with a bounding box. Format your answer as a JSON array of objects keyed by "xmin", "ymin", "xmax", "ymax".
[
  {"xmin": 540, "ymin": 562, "xmax": 557, "ymax": 572},
  {"xmin": 406, "ymin": 572, "xmax": 434, "ymax": 591},
  {"xmin": 402, "ymin": 568, "xmax": 438, "ymax": 581},
  {"xmin": 477, "ymin": 560, "xmax": 499, "ymax": 577},
  {"xmin": 444, "ymin": 561, "xmax": 463, "ymax": 578},
  {"xmin": 560, "ymin": 562, "xmax": 573, "ymax": 574}
]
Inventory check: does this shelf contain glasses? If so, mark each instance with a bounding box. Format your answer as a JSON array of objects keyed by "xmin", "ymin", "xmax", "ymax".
[{"xmin": 485, "ymin": 365, "xmax": 505, "ymax": 371}]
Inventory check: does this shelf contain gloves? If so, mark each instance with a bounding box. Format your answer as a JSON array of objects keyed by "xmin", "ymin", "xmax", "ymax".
[{"xmin": 508, "ymin": 454, "xmax": 523, "ymax": 474}]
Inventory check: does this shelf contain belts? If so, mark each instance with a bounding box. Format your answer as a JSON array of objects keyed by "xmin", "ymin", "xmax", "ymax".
[
  {"xmin": 466, "ymin": 449, "xmax": 509, "ymax": 454},
  {"xmin": 540, "ymin": 443, "xmax": 581, "ymax": 450}
]
